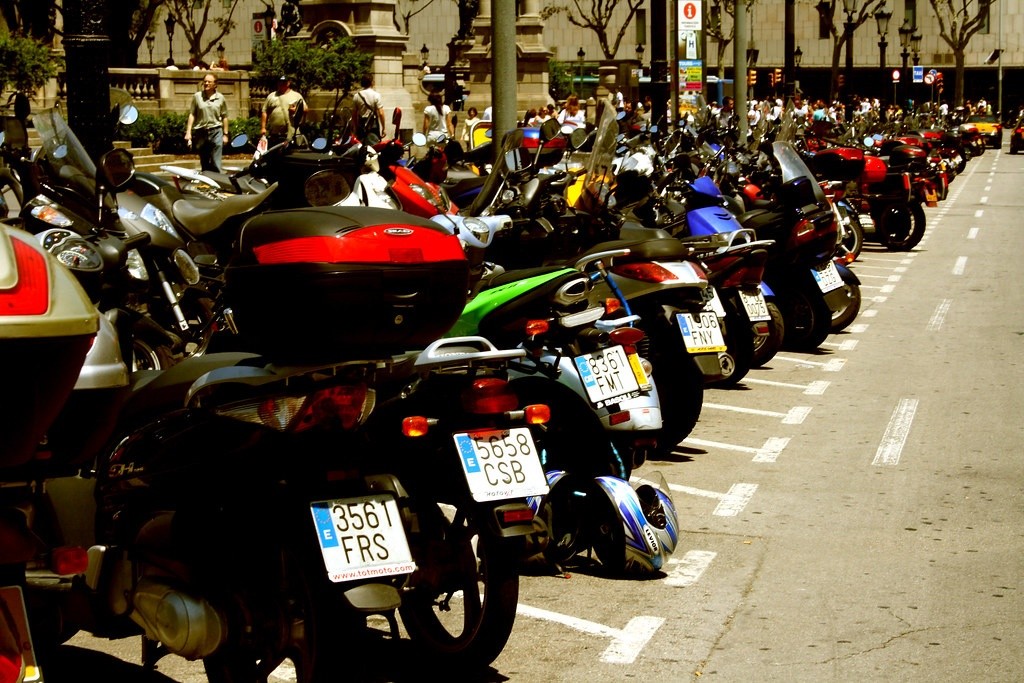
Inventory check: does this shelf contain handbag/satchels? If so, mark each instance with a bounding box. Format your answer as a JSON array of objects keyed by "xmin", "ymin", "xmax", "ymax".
[{"xmin": 252, "ymin": 135, "xmax": 268, "ymax": 160}]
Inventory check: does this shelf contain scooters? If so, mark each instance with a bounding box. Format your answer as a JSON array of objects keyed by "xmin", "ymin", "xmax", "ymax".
[
  {"xmin": 788, "ymin": 107, "xmax": 986, "ymax": 250},
  {"xmin": 0, "ymin": 98, "xmax": 863, "ymax": 682}
]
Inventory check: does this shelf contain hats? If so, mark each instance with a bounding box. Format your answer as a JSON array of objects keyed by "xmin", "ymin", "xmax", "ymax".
[{"xmin": 275, "ymin": 74, "xmax": 290, "ymax": 83}]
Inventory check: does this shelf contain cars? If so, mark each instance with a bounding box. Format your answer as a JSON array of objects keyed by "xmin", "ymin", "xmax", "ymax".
[
  {"xmin": 958, "ymin": 114, "xmax": 1002, "ymax": 149},
  {"xmin": 1010, "ymin": 115, "xmax": 1024, "ymax": 154}
]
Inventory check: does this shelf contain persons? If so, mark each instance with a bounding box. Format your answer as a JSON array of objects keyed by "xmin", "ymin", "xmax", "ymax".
[
  {"xmin": 423, "ymin": 91, "xmax": 454, "ymax": 160},
  {"xmin": 460, "ymin": 85, "xmax": 990, "ymax": 151},
  {"xmin": 259, "ymin": 74, "xmax": 308, "ymax": 150},
  {"xmin": 184, "ymin": 73, "xmax": 229, "ymax": 174},
  {"xmin": 164, "ymin": 57, "xmax": 230, "ymax": 72},
  {"xmin": 350, "ymin": 74, "xmax": 386, "ymax": 144}
]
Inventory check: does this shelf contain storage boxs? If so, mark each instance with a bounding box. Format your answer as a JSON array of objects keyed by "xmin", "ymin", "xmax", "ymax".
[
  {"xmin": 234, "ymin": 204, "xmax": 469, "ymax": 364},
  {"xmin": 273, "ymin": 149, "xmax": 355, "ymax": 211}
]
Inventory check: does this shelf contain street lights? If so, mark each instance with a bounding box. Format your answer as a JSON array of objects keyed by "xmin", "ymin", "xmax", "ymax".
[
  {"xmin": 795, "ymin": 46, "xmax": 803, "ymax": 73},
  {"xmin": 897, "ymin": 18, "xmax": 918, "ymax": 81},
  {"xmin": 842, "ymin": 0, "xmax": 857, "ymax": 95},
  {"xmin": 873, "ymin": 0, "xmax": 894, "ymax": 72},
  {"xmin": 635, "ymin": 43, "xmax": 645, "ymax": 69},
  {"xmin": 910, "ymin": 34, "xmax": 922, "ymax": 66},
  {"xmin": 577, "ymin": 46, "xmax": 586, "ymax": 98},
  {"xmin": 146, "ymin": 32, "xmax": 155, "ymax": 65},
  {"xmin": 164, "ymin": 13, "xmax": 176, "ymax": 66},
  {"xmin": 263, "ymin": 5, "xmax": 275, "ymax": 45},
  {"xmin": 893, "ymin": 71, "xmax": 900, "ymax": 106}
]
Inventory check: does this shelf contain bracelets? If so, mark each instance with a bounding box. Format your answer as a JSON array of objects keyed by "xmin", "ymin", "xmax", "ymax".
[{"xmin": 223, "ymin": 133, "xmax": 229, "ymax": 135}]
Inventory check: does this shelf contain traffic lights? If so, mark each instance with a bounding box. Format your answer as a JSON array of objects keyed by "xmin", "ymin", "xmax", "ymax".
[
  {"xmin": 768, "ymin": 69, "xmax": 781, "ymax": 87},
  {"xmin": 748, "ymin": 70, "xmax": 756, "ymax": 86}
]
